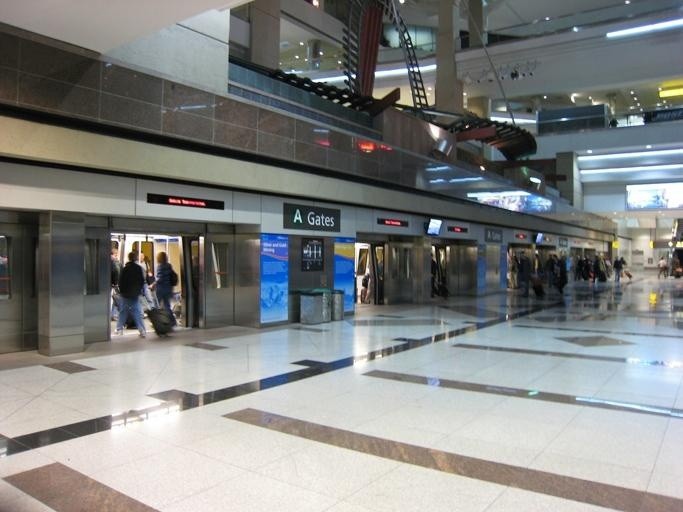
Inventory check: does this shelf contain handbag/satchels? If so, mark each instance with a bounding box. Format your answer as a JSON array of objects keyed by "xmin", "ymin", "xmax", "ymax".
[
  {"xmin": 532, "ymin": 277, "xmax": 542, "ymax": 285},
  {"xmin": 170, "ymin": 270, "xmax": 177, "ymax": 285},
  {"xmin": 147, "ymin": 274, "xmax": 155, "ymax": 285}
]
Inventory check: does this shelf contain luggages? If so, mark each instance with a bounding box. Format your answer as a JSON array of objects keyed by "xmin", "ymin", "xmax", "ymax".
[
  {"xmin": 599, "ymin": 272, "xmax": 607, "ymax": 282},
  {"xmin": 145, "ymin": 307, "xmax": 172, "ymax": 336},
  {"xmin": 532, "ymin": 285, "xmax": 543, "ymax": 296},
  {"xmin": 438, "ymin": 284, "xmax": 448, "ymax": 299}
]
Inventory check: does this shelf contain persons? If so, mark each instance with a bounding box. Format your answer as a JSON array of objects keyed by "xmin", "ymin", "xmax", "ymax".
[
  {"xmin": 656, "ymin": 256, "xmax": 666, "ymax": 279},
  {"xmin": 108, "ymin": 246, "xmax": 183, "ymax": 338},
  {"xmin": 506, "ymin": 236, "xmax": 628, "ymax": 299},
  {"xmin": 673, "ymin": 255, "xmax": 680, "ymax": 278},
  {"xmin": 431, "ymin": 253, "xmax": 438, "ymax": 296}
]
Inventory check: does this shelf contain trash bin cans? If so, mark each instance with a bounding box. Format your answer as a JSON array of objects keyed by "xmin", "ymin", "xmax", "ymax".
[{"xmin": 299, "ymin": 288, "xmax": 344, "ymax": 325}]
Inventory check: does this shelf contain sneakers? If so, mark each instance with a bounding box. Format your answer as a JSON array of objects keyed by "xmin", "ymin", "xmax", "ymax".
[
  {"xmin": 522, "ymin": 293, "xmax": 529, "ymax": 297},
  {"xmin": 112, "ymin": 317, "xmax": 119, "ymax": 321},
  {"xmin": 139, "ymin": 333, "xmax": 146, "ymax": 337},
  {"xmin": 115, "ymin": 330, "xmax": 123, "ymax": 335}
]
